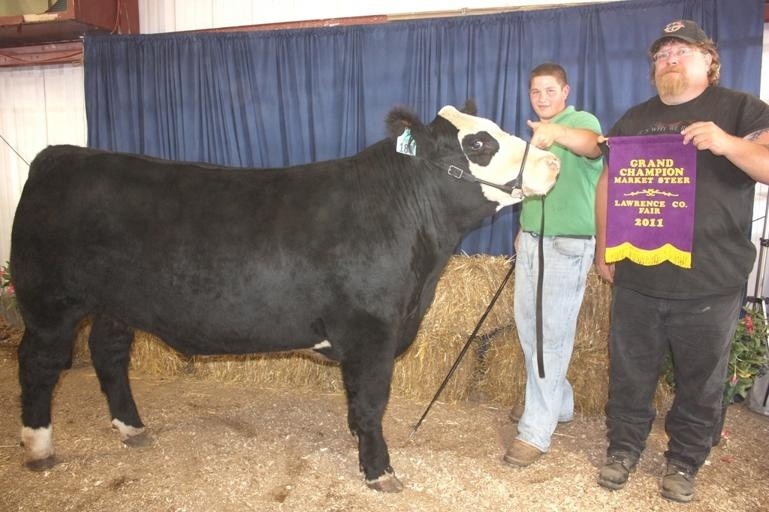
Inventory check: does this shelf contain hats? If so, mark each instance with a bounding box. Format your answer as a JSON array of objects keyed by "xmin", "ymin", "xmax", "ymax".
[{"xmin": 650, "ymin": 19, "xmax": 707, "ymax": 47}]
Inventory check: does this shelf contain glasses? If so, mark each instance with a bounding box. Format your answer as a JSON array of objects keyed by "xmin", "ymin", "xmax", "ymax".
[{"xmin": 652, "ymin": 46, "xmax": 692, "ymax": 63}]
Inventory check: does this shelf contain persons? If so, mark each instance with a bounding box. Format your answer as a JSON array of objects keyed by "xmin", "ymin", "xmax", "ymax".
[
  {"xmin": 502, "ymin": 62, "xmax": 604, "ymax": 466},
  {"xmin": 595, "ymin": 18, "xmax": 769, "ymax": 502}
]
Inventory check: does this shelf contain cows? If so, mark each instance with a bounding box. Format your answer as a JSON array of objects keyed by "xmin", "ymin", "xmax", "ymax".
[{"xmin": 9, "ymin": 99, "xmax": 562, "ymax": 495}]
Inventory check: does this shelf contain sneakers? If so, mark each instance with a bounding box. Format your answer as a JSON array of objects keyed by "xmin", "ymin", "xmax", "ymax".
[
  {"xmin": 509, "ymin": 401, "xmax": 525, "ymax": 422},
  {"xmin": 502, "ymin": 437, "xmax": 542, "ymax": 468},
  {"xmin": 596, "ymin": 452, "xmax": 637, "ymax": 489},
  {"xmin": 659, "ymin": 460, "xmax": 699, "ymax": 503}
]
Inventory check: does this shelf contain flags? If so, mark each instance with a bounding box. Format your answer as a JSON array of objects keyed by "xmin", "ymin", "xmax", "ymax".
[{"xmin": 604, "ymin": 132, "xmax": 699, "ymax": 269}]
